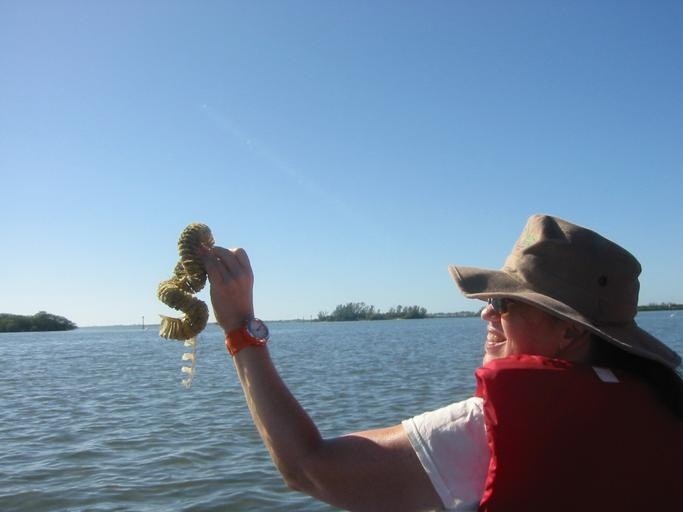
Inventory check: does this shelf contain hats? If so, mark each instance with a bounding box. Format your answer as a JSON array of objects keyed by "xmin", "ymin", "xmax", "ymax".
[{"xmin": 449, "ymin": 214, "xmax": 683, "ymax": 370}]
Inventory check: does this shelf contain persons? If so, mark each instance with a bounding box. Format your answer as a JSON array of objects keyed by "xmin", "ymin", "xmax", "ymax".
[{"xmin": 203, "ymin": 210, "xmax": 682, "ymax": 512}]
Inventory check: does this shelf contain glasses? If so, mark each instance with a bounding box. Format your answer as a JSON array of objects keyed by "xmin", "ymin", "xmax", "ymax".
[{"xmin": 488, "ymin": 298, "xmax": 516, "ymax": 316}]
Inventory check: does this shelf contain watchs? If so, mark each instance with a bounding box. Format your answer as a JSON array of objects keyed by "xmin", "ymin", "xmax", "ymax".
[{"xmin": 224, "ymin": 318, "xmax": 270, "ymax": 355}]
